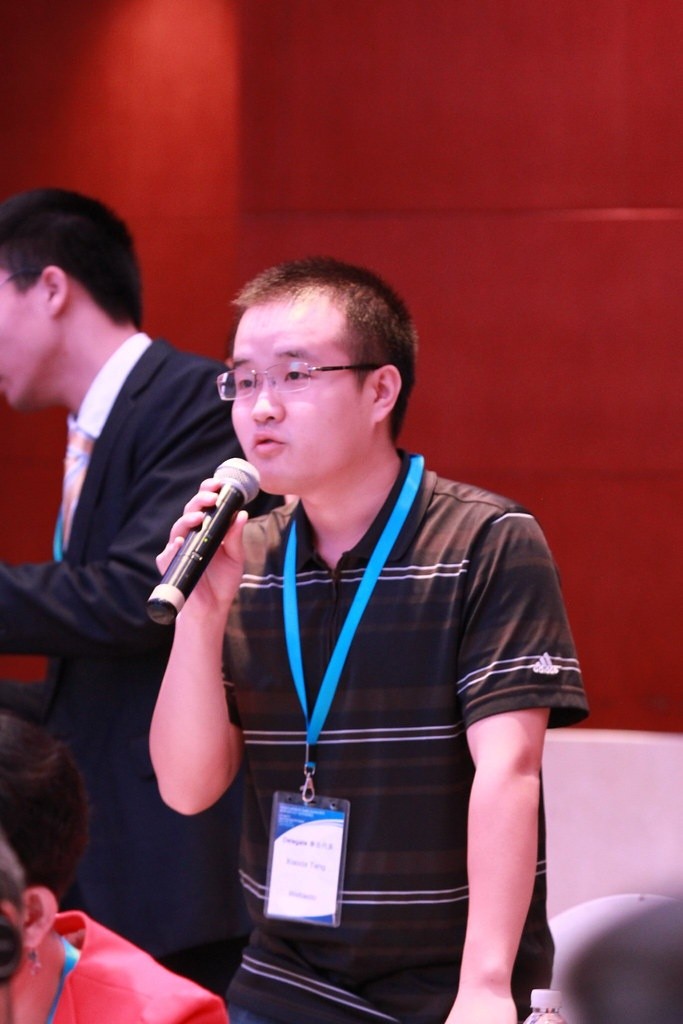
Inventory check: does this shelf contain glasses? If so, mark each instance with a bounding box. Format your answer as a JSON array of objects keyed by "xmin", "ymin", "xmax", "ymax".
[{"xmin": 214, "ymin": 359, "xmax": 383, "ymax": 400}]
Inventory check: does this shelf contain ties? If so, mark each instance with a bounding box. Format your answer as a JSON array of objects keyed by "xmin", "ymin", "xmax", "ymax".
[{"xmin": 59, "ymin": 428, "xmax": 97, "ymax": 554}]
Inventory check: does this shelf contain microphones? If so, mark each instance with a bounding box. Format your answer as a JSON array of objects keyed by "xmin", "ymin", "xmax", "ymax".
[{"xmin": 147, "ymin": 458, "xmax": 260, "ymax": 624}]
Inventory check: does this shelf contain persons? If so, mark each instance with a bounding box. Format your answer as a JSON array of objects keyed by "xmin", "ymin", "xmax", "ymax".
[
  {"xmin": 149, "ymin": 256, "xmax": 591, "ymax": 1024},
  {"xmin": 0, "ymin": 189, "xmax": 286, "ymax": 998},
  {"xmin": 0, "ymin": 717, "xmax": 231, "ymax": 1024}
]
